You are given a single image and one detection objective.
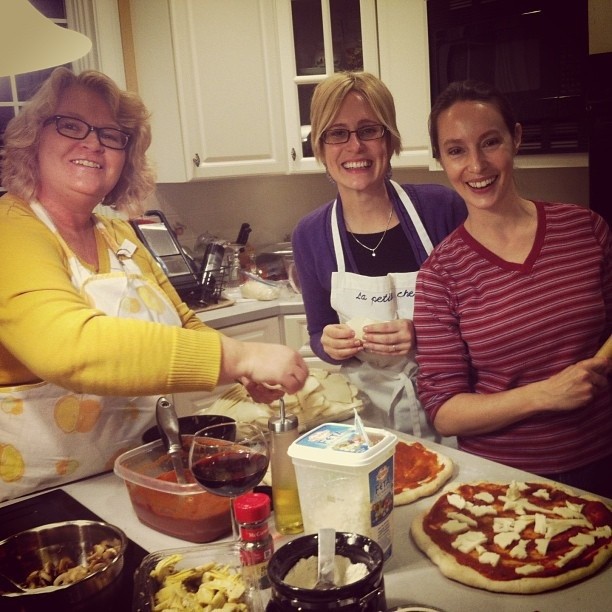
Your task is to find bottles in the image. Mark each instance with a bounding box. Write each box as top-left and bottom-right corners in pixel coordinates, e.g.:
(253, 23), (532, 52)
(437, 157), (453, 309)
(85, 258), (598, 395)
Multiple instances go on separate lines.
(230, 493), (275, 588)
(267, 396), (303, 536)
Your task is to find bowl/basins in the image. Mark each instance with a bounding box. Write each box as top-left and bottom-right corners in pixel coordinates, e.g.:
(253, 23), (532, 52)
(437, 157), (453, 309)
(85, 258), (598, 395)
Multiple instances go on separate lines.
(1, 519), (128, 611)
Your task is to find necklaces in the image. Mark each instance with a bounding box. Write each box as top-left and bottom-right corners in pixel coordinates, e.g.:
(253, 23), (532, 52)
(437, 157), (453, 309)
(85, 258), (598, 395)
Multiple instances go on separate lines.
(343, 204), (395, 256)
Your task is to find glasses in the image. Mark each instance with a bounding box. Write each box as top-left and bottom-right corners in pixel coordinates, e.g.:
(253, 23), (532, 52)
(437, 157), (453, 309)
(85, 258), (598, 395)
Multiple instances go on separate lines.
(319, 125), (390, 144)
(43, 115), (133, 150)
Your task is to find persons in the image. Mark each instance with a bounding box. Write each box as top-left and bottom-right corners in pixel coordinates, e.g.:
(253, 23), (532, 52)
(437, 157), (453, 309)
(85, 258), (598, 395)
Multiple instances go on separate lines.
(1, 63), (310, 502)
(289, 71), (467, 452)
(412, 83), (610, 503)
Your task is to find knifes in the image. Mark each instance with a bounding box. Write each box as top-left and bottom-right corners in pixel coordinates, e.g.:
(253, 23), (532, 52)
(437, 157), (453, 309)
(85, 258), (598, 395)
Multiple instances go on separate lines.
(240, 227), (251, 243)
(235, 222), (250, 244)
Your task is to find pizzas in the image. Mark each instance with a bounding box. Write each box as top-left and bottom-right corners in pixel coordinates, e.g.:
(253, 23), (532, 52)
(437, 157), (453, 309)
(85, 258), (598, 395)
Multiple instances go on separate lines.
(393, 438), (454, 507)
(409, 479), (612, 594)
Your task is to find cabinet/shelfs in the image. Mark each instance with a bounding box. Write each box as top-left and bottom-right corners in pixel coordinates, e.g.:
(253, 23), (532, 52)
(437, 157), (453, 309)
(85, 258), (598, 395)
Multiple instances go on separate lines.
(216, 316), (282, 349)
(282, 313), (313, 356)
(276, 0), (431, 176)
(119, 1), (290, 183)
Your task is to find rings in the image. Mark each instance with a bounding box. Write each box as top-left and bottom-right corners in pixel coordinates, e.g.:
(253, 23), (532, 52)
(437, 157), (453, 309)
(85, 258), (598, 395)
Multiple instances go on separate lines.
(393, 345), (397, 352)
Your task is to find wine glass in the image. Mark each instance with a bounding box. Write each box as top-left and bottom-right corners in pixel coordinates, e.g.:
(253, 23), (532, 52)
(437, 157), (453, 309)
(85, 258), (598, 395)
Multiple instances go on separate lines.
(186, 419), (270, 542)
